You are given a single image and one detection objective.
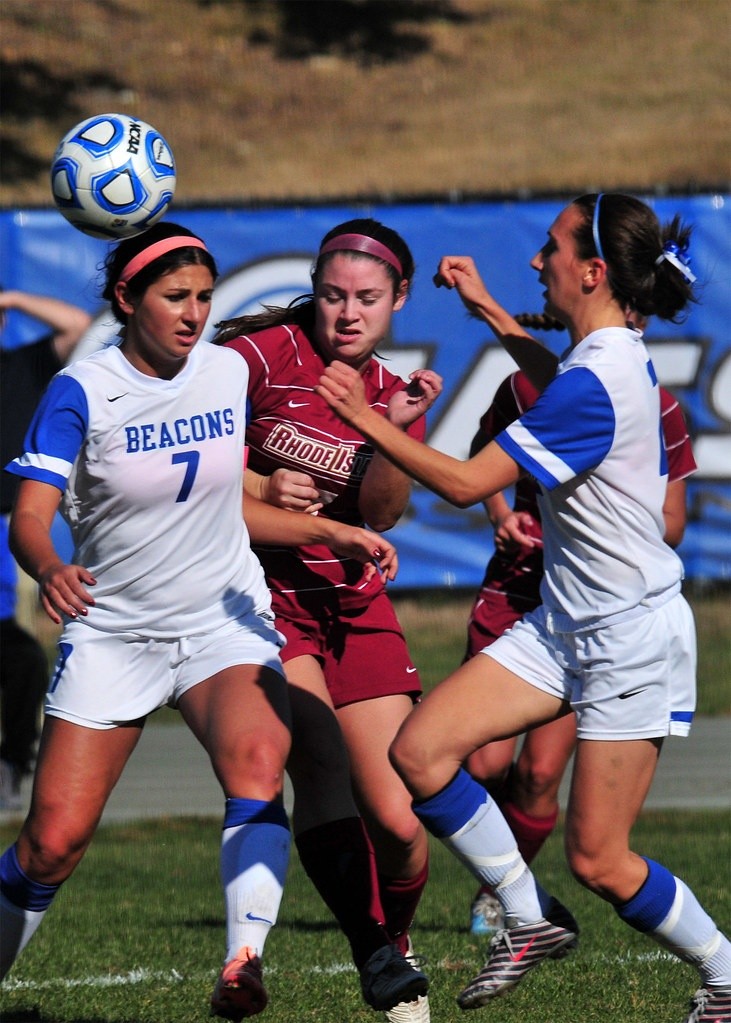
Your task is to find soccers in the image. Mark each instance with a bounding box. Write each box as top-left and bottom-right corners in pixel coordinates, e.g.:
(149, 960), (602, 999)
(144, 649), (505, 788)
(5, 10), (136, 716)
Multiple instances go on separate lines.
(49, 113), (178, 242)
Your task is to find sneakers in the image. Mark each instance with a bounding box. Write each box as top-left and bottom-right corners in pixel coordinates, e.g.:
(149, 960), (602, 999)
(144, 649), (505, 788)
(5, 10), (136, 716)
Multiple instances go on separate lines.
(455, 918), (577, 1010)
(469, 893), (506, 933)
(358, 934), (431, 1022)
(206, 946), (268, 1022)
(686, 984), (731, 1023)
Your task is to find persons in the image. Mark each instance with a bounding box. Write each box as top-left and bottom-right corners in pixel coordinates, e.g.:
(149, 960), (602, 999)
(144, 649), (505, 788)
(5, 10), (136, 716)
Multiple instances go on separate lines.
(0, 291), (93, 794)
(0, 220), (297, 1020)
(316, 191), (731, 1023)
(458, 303), (697, 937)
(209, 220), (443, 1023)
